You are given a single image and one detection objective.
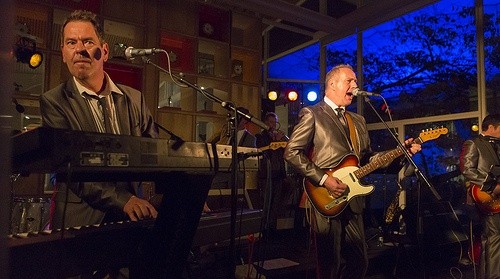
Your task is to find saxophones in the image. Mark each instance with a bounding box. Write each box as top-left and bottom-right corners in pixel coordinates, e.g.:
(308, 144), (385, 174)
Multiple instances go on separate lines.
(379, 176), (404, 225)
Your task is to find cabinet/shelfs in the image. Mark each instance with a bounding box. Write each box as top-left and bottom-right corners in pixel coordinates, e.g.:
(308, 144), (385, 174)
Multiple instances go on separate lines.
(156, 0), (263, 142)
(14, 0), (148, 199)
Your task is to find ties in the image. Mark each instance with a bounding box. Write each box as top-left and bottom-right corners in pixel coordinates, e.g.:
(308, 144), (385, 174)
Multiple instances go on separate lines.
(92, 95), (115, 135)
(335, 108), (354, 149)
(489, 141), (500, 161)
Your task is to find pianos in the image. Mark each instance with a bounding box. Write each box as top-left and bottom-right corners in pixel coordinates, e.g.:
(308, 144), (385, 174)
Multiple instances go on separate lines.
(0, 123), (273, 279)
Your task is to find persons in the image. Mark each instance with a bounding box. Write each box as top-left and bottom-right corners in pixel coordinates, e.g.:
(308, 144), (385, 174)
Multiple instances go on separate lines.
(255, 112), (288, 243)
(283, 64), (421, 279)
(39, 9), (209, 230)
(463, 113), (500, 279)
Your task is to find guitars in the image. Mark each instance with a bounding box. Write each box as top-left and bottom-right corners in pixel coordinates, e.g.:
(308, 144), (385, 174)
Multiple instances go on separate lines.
(248, 141), (292, 156)
(303, 126), (449, 218)
(471, 161), (500, 215)
(467, 236), (485, 266)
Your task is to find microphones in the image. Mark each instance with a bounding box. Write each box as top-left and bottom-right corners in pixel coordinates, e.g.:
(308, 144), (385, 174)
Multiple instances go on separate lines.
(124, 47), (165, 59)
(483, 136), (500, 141)
(352, 88), (380, 96)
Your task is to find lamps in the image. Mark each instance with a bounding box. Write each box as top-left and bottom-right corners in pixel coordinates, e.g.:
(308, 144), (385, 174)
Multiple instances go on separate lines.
(15, 45), (44, 69)
(266, 87), (281, 103)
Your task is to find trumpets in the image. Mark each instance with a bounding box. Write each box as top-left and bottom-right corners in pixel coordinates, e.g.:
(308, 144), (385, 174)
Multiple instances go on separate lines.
(271, 121), (295, 144)
